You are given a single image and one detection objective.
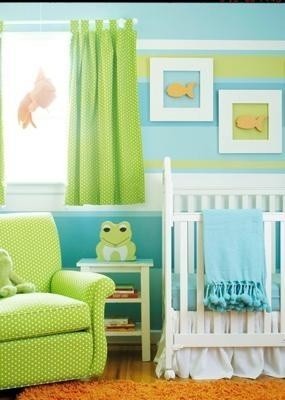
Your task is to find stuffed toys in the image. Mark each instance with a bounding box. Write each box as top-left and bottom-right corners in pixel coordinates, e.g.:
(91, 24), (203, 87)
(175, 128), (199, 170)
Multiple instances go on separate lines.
(96, 221), (138, 261)
(16, 70), (56, 129)
(0, 249), (36, 297)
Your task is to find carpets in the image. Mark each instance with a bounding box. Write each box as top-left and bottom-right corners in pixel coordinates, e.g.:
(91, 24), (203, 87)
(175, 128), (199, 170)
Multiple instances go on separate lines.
(14, 379), (285, 400)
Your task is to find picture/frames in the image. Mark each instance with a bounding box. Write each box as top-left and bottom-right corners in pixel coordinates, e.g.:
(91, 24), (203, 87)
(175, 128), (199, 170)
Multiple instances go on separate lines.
(150, 58), (283, 154)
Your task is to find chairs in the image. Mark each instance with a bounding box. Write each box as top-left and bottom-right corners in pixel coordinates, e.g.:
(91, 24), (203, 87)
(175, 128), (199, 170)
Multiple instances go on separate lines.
(0, 212), (115, 392)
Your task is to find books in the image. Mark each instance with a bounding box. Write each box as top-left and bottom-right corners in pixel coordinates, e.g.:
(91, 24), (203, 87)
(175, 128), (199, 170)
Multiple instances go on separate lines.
(106, 286), (139, 298)
(104, 314), (137, 332)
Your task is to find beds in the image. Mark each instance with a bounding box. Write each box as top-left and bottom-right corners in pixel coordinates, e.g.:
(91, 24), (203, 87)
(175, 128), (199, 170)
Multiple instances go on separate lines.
(161, 157), (285, 380)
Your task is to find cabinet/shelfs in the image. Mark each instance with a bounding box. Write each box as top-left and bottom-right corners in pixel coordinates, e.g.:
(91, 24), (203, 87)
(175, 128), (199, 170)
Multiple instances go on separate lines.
(75, 258), (153, 362)
